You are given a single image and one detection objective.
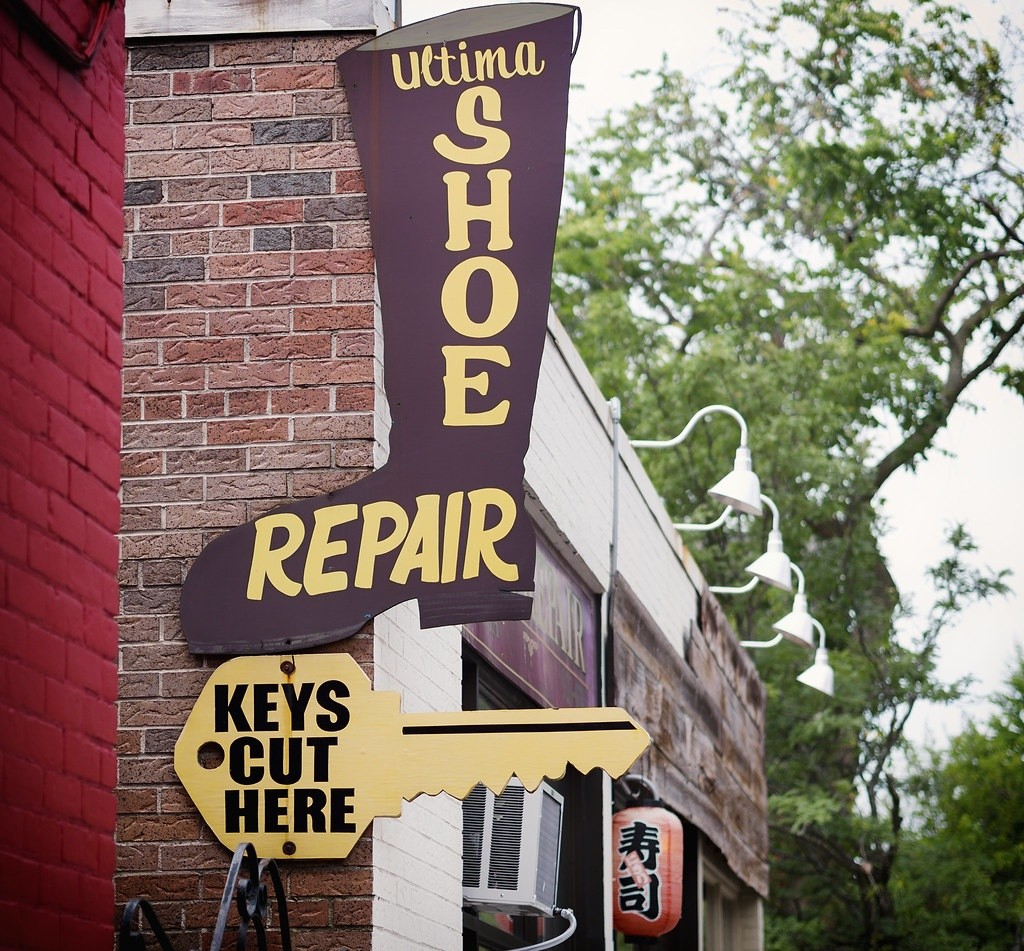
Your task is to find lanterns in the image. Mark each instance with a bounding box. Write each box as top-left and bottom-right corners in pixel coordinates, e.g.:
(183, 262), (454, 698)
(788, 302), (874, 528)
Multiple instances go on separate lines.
(612, 807), (684, 942)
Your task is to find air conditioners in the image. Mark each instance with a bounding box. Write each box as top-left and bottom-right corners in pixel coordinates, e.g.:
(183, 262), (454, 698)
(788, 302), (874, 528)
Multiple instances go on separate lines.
(462, 776), (565, 918)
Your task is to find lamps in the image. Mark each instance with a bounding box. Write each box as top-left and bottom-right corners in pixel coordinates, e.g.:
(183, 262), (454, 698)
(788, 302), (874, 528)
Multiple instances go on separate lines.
(628, 404), (835, 699)
